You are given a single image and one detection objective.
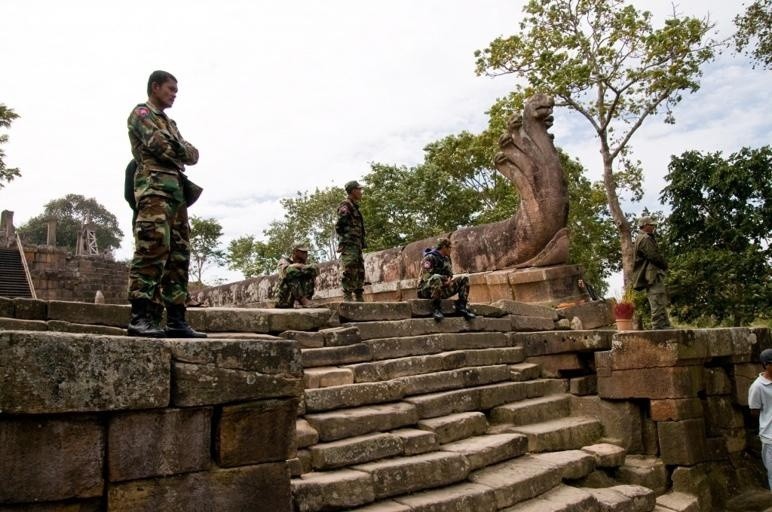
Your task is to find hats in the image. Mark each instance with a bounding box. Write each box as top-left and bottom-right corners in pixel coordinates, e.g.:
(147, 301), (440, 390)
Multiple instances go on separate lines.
(292, 241), (310, 252)
(437, 237), (453, 248)
(345, 180), (364, 191)
(760, 348), (772, 367)
(639, 217), (657, 227)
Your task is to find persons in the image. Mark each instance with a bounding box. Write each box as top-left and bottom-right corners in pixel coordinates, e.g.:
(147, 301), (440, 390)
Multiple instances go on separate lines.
(274, 241), (323, 308)
(630, 215), (675, 329)
(416, 237), (476, 319)
(335, 180), (368, 301)
(747, 347), (772, 490)
(126, 69), (208, 338)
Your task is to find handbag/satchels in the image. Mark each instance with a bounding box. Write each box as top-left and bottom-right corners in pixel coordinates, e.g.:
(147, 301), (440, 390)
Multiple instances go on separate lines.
(179, 172), (203, 207)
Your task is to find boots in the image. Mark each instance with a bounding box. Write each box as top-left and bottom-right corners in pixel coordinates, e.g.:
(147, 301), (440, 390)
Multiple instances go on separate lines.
(431, 298), (445, 319)
(455, 299), (475, 320)
(125, 297), (208, 339)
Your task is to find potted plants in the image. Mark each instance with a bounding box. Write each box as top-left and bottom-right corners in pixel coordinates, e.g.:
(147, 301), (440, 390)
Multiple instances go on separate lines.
(611, 301), (634, 330)
